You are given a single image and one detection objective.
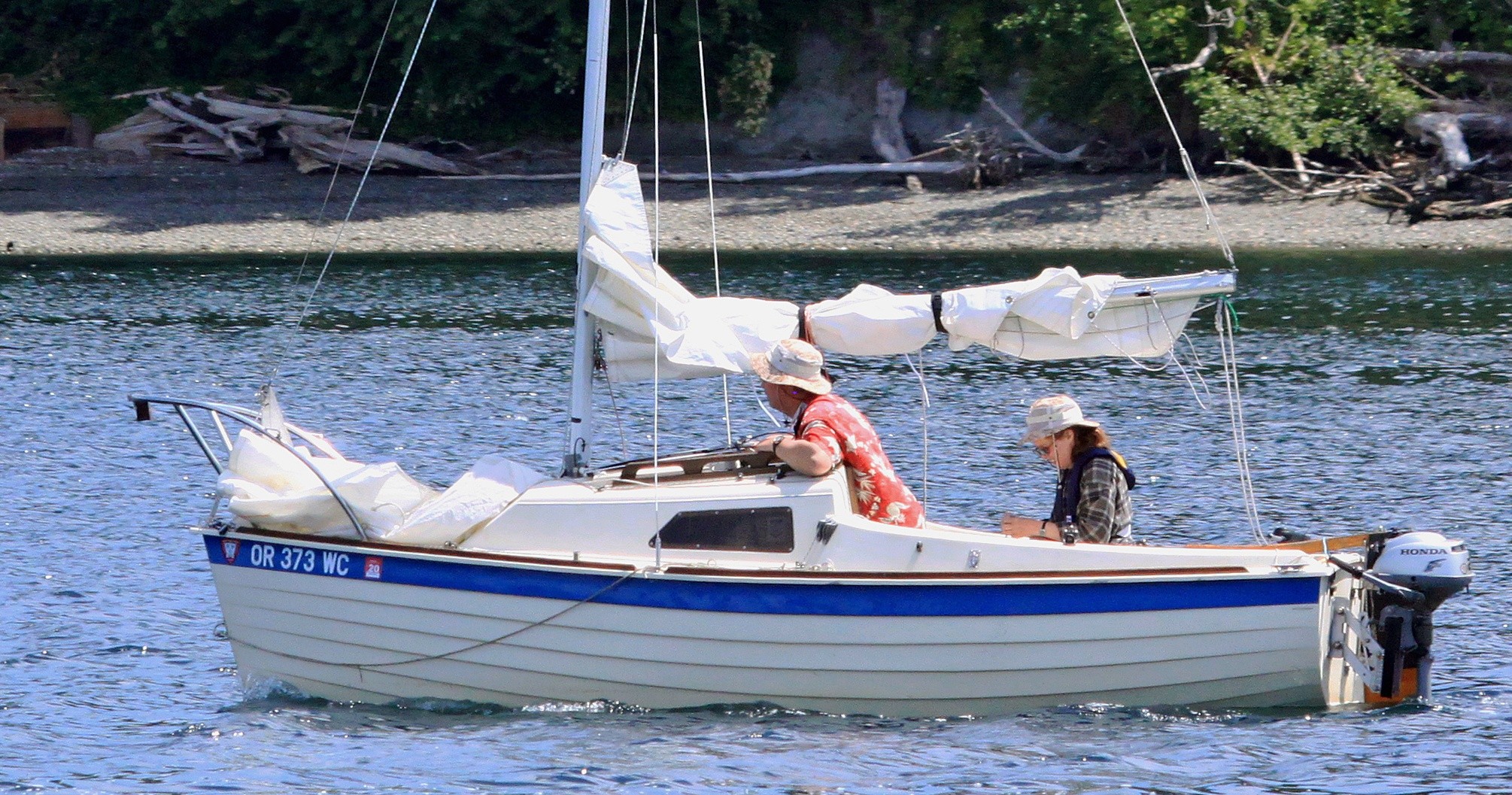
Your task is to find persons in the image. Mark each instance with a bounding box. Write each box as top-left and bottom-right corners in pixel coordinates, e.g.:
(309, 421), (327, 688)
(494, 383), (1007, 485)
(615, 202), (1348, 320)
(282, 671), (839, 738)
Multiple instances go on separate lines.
(752, 337), (925, 529)
(1000, 395), (1133, 544)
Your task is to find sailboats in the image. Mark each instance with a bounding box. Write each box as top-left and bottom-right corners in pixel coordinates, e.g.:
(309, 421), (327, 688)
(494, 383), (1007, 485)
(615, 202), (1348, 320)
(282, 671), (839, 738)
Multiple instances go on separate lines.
(127, 0), (1475, 715)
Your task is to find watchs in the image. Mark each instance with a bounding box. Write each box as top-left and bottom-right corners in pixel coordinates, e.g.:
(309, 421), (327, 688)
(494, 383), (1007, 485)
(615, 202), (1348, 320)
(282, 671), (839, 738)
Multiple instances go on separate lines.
(773, 434), (785, 455)
(1039, 521), (1048, 537)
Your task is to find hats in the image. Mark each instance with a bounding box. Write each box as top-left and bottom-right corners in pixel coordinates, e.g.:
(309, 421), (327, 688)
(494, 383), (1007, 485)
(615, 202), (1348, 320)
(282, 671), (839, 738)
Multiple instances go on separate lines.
(748, 338), (832, 396)
(1017, 394), (1101, 446)
